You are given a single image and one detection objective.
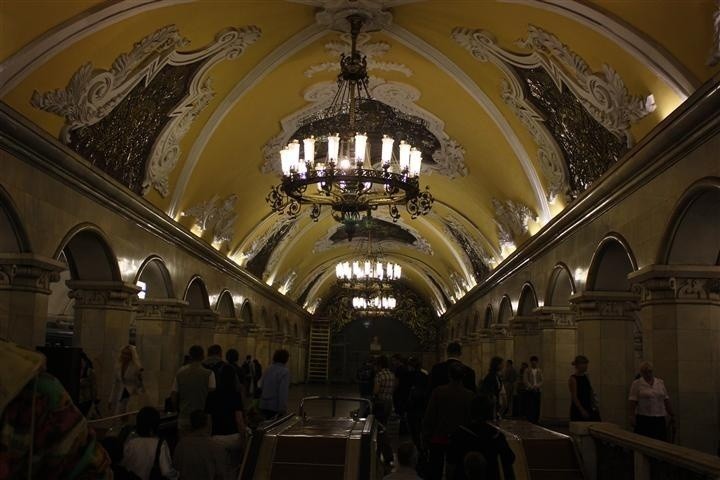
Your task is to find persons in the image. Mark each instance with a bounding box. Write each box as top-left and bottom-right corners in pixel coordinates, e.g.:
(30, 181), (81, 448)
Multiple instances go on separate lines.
(108, 343), (291, 480)
(625, 361), (675, 442)
(568, 356), (602, 422)
(358, 342), (543, 480)
